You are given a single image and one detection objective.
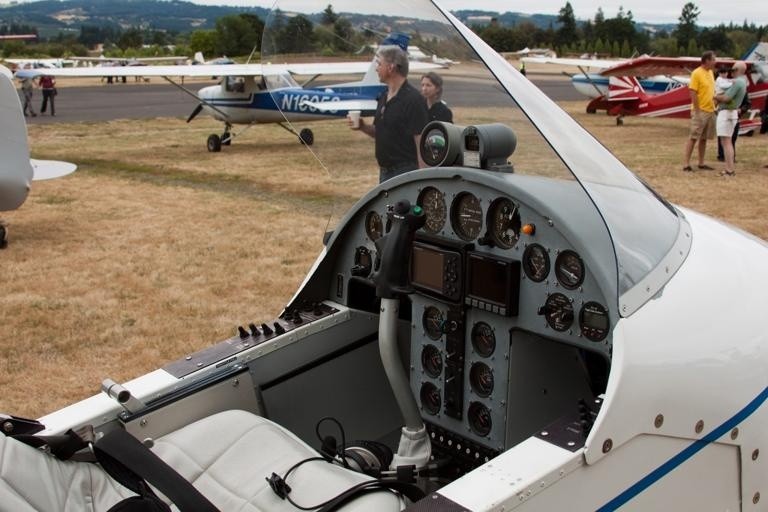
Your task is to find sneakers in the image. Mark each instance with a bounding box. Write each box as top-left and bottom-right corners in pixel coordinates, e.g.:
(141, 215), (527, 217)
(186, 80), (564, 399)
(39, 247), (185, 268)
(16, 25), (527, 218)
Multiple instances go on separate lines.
(684, 166), (692, 172)
(699, 164), (713, 169)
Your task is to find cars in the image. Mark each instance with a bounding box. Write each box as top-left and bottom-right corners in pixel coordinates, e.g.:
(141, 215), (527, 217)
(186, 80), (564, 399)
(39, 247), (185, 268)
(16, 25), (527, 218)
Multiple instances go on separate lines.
(7, 56), (73, 72)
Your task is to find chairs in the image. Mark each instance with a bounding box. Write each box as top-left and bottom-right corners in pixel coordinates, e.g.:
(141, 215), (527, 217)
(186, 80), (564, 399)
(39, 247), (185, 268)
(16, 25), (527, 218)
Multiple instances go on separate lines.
(0, 408), (407, 512)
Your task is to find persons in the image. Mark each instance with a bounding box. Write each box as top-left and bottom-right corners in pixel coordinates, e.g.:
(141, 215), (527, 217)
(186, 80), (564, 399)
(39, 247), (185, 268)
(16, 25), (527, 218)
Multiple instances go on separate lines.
(416, 71), (455, 125)
(99, 60), (141, 85)
(716, 92), (748, 164)
(37, 65), (58, 117)
(681, 51), (719, 172)
(713, 60), (748, 178)
(345, 44), (433, 185)
(18, 64), (39, 118)
(714, 66), (733, 116)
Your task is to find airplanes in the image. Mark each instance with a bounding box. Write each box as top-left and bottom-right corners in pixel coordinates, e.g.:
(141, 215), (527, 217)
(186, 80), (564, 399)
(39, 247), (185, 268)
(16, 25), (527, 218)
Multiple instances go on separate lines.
(581, 51), (768, 138)
(523, 40), (768, 99)
(75, 53), (190, 83)
(13, 54), (451, 152)
(0, 0), (768, 512)
(0, 31), (80, 248)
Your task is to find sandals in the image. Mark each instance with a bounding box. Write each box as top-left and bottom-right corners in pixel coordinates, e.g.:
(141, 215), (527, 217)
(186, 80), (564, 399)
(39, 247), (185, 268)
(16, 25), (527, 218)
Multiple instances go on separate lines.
(717, 170), (736, 177)
